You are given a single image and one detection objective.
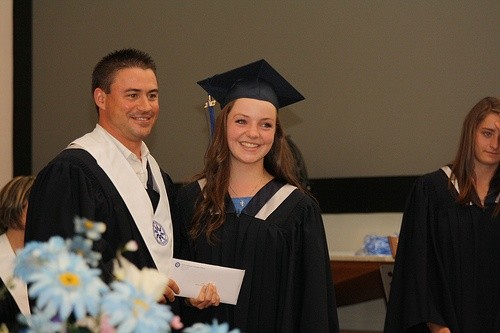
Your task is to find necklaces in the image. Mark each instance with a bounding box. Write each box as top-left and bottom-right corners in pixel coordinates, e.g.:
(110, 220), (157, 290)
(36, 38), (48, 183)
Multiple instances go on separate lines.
(226, 181), (264, 207)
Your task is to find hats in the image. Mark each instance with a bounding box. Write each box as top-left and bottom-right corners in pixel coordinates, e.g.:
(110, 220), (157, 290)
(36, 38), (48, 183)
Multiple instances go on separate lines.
(196, 59), (307, 111)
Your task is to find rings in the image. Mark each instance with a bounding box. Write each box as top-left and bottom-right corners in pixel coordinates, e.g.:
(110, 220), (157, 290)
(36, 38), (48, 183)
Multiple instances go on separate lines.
(196, 304), (204, 309)
(192, 303), (199, 307)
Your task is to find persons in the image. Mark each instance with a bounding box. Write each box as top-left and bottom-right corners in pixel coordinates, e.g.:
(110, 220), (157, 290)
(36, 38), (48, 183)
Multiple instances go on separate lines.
(384, 96), (500, 333)
(167, 58), (341, 333)
(0, 176), (38, 333)
(21, 47), (221, 333)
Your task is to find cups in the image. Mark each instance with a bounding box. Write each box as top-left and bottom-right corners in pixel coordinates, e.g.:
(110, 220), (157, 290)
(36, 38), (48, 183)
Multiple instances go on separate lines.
(387, 235), (398, 257)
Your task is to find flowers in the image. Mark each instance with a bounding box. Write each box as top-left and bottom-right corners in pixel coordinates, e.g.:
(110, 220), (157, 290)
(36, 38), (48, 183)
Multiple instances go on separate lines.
(6, 218), (240, 333)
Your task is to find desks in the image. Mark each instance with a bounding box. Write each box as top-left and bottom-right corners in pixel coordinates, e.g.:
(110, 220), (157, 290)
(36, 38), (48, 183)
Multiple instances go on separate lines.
(329, 253), (394, 306)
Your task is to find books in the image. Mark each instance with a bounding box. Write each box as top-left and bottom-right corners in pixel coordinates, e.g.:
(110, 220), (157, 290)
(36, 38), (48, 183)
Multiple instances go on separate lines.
(387, 235), (398, 259)
